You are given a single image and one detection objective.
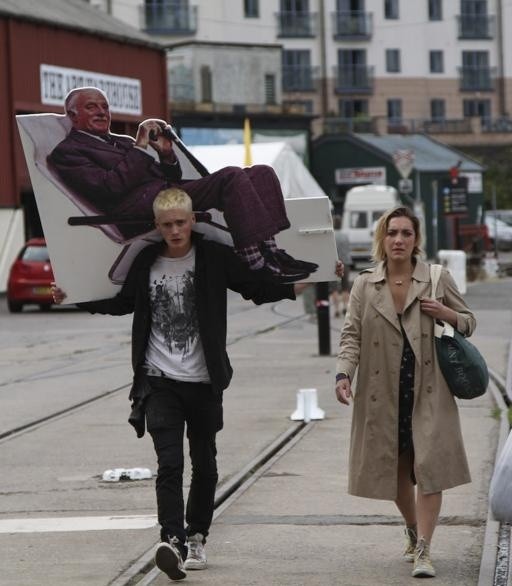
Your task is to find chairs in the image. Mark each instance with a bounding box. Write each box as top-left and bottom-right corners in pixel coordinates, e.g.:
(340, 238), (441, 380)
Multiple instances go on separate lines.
(14, 111), (280, 291)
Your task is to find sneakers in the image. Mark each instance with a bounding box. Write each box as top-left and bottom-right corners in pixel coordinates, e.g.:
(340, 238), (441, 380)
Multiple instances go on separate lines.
(403, 522), (435, 578)
(185, 533), (207, 569)
(153, 538), (187, 580)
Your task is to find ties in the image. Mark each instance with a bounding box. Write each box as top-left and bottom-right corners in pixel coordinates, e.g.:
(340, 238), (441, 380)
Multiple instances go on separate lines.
(101, 134), (130, 152)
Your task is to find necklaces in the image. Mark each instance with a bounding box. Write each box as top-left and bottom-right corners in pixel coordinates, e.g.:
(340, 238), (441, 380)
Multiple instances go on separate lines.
(394, 276), (413, 285)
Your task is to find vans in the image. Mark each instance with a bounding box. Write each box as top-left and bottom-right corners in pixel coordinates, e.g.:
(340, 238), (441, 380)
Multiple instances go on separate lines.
(341, 184), (402, 269)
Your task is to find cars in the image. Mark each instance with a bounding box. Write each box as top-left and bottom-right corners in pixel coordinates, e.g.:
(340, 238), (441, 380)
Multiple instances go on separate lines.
(5, 239), (56, 314)
(484, 213), (512, 252)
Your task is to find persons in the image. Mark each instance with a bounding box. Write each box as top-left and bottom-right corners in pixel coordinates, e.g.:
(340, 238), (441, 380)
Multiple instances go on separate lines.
(334, 205), (476, 579)
(46, 88), (319, 284)
(50, 187), (346, 581)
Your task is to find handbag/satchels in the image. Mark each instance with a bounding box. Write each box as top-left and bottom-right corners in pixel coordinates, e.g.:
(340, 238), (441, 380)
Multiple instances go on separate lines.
(434, 319), (488, 399)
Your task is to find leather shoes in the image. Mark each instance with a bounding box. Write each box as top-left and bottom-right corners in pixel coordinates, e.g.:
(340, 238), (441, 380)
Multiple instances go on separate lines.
(244, 249), (318, 283)
